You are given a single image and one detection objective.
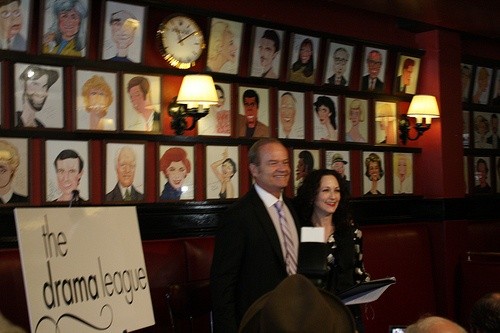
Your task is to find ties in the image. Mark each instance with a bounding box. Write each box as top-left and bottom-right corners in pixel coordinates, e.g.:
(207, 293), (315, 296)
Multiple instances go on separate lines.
(274, 201), (297, 276)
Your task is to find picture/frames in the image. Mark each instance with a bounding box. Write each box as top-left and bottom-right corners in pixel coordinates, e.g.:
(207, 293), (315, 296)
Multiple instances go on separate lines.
(460, 62), (500, 196)
(0, 0), (423, 206)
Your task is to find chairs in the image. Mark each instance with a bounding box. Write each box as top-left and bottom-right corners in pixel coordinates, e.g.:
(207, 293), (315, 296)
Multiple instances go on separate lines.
(165, 281), (213, 333)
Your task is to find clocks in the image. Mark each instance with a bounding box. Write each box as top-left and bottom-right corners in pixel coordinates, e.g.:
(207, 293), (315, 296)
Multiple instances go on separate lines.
(154, 15), (205, 69)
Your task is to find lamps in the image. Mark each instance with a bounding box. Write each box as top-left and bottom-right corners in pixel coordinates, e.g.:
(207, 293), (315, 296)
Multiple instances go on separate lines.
(399, 95), (440, 145)
(168, 73), (218, 135)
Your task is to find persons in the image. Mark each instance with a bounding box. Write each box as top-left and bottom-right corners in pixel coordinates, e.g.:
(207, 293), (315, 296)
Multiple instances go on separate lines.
(239, 274), (356, 333)
(468, 293), (500, 333)
(209, 137), (307, 333)
(406, 316), (467, 333)
(293, 168), (370, 333)
